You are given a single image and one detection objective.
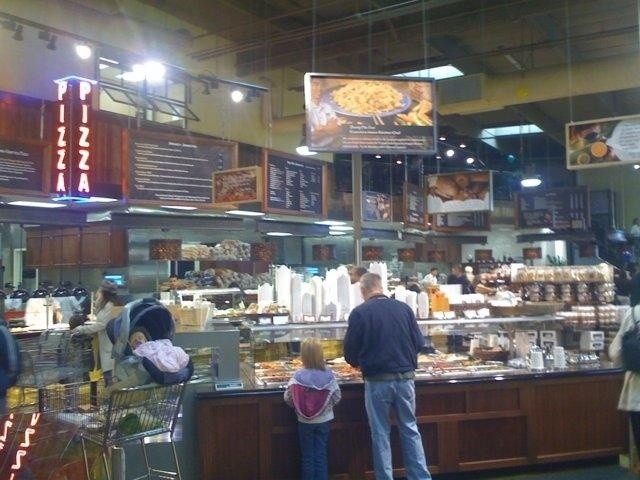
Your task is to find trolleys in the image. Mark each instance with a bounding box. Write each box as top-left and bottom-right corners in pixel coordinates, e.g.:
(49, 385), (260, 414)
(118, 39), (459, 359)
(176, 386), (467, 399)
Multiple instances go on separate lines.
(0, 327), (189, 480)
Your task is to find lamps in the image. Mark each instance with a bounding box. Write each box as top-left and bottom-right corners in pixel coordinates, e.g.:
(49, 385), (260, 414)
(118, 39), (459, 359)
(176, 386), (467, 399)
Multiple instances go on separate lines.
(522, 241), (542, 260)
(1, 10), (269, 103)
(251, 234), (279, 261)
(578, 240), (599, 258)
(427, 244), (446, 263)
(312, 236), (337, 261)
(474, 244), (493, 261)
(521, 124), (541, 188)
(362, 238), (384, 261)
(398, 242), (416, 261)
(148, 226), (181, 261)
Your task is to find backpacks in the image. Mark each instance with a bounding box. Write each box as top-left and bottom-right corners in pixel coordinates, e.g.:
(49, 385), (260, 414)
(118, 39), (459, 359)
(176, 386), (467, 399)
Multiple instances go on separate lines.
(623, 310), (640, 371)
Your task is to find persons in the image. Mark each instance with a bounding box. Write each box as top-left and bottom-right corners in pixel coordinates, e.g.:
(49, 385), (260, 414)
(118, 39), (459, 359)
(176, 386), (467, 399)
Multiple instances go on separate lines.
(422, 267), (438, 284)
(608, 270), (640, 477)
(348, 266), (370, 308)
(0, 278), (125, 409)
(310, 74), (345, 149)
(631, 218), (640, 237)
(444, 262), (476, 353)
(0, 292), (23, 416)
(219, 282), (261, 343)
(343, 271), (432, 480)
(284, 336), (343, 479)
(163, 272), (190, 301)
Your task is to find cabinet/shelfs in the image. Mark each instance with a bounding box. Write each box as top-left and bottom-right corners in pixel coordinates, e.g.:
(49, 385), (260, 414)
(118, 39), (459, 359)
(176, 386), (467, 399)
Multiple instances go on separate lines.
(23, 225), (127, 271)
(193, 316), (629, 480)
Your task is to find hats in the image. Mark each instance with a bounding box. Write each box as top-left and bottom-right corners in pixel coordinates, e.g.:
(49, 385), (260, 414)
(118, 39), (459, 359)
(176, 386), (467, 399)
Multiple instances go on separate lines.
(100, 280), (118, 294)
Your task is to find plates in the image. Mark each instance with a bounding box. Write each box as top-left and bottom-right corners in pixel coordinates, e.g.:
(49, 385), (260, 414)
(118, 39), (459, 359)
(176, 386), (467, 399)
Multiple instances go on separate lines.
(321, 83), (413, 119)
(577, 153), (591, 165)
(591, 141), (608, 158)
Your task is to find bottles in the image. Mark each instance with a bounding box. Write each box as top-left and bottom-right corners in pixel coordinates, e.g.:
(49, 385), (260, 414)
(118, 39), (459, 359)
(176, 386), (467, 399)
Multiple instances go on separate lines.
(0, 281), (90, 314)
(477, 259), (496, 274)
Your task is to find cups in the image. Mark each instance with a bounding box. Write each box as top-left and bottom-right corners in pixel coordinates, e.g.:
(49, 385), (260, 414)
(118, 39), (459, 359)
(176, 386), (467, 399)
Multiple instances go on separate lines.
(553, 347), (565, 367)
(258, 260), (431, 323)
(530, 352), (544, 370)
(490, 335), (498, 347)
(470, 339), (480, 354)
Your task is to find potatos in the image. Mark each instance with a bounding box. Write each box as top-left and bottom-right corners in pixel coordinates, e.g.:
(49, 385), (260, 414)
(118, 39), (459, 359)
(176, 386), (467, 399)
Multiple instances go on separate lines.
(436, 173), (489, 201)
